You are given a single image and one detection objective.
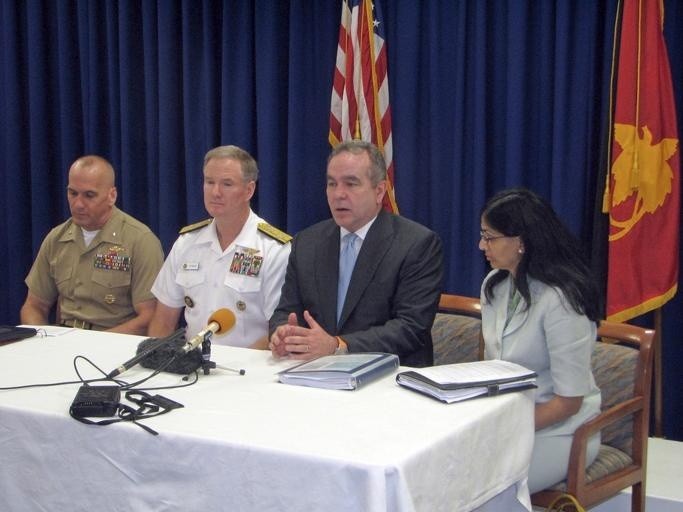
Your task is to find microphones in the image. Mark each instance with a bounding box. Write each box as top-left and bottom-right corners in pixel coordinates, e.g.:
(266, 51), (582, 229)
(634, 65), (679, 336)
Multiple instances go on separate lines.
(174, 308), (235, 360)
(107, 327), (186, 377)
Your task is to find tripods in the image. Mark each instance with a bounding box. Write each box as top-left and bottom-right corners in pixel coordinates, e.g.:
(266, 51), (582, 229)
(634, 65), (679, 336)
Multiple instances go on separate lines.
(183, 343), (245, 380)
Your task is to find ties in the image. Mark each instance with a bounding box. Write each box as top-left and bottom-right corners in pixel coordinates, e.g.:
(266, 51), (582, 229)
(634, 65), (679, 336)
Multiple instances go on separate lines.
(335, 233), (359, 325)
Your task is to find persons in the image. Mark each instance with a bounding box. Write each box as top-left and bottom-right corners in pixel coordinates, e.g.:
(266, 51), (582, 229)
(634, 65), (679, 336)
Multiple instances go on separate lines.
(19, 154), (165, 335)
(268, 141), (444, 368)
(147, 145), (292, 350)
(478, 188), (601, 493)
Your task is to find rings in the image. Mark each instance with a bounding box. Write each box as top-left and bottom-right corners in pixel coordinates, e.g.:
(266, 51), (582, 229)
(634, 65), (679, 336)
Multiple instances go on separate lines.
(307, 345), (309, 351)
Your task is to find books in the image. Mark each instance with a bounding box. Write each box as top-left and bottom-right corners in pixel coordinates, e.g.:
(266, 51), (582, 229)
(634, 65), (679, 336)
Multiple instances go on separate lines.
(273, 352), (539, 404)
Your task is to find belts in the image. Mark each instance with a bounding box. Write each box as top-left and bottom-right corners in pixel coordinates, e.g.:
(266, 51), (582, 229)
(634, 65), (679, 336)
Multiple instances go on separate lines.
(64, 318), (109, 332)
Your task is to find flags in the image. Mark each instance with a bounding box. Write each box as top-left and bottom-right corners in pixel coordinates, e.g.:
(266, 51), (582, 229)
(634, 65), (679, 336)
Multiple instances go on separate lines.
(601, 0), (681, 323)
(328, 1), (401, 216)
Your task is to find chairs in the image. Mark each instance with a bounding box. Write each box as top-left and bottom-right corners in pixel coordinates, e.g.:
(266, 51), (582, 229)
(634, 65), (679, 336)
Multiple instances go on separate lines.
(430, 294), (486, 376)
(530, 325), (658, 512)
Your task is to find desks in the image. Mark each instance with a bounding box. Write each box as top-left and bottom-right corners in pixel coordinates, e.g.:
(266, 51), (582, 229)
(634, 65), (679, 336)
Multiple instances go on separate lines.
(0, 324), (535, 512)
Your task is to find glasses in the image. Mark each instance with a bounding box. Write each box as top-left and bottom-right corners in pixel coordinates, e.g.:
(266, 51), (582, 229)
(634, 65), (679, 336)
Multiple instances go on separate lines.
(480, 230), (506, 244)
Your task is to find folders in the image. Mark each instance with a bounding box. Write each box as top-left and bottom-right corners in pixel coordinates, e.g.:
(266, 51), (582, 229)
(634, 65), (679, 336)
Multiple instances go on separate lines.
(271, 347), (539, 407)
